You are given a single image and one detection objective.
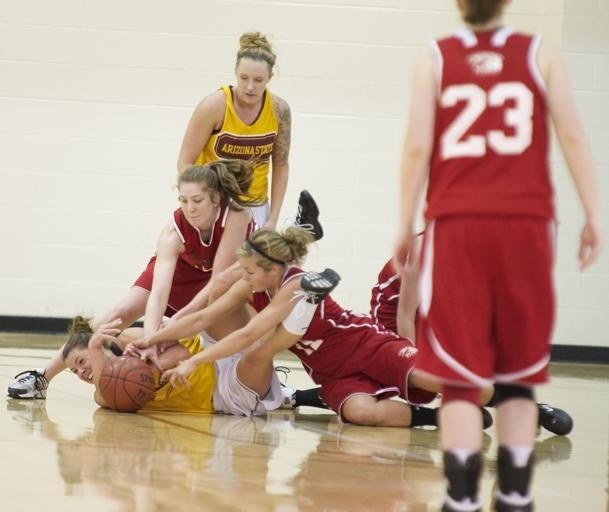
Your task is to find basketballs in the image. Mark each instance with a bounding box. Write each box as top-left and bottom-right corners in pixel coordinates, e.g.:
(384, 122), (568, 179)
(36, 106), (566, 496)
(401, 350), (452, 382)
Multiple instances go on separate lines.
(98, 355), (155, 411)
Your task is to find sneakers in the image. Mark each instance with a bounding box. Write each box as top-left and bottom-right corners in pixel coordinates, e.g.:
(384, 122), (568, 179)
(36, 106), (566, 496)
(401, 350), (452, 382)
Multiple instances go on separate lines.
(8, 367), (49, 399)
(278, 387), (296, 409)
(433, 405), (493, 429)
(538, 404), (573, 435)
(295, 191), (323, 241)
(300, 268), (340, 304)
(442, 483), (535, 512)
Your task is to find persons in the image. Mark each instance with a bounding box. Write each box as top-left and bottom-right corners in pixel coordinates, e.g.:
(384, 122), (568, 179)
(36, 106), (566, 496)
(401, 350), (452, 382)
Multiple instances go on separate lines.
(393, 0), (601, 512)
(276, 227), (425, 409)
(6, 160), (255, 400)
(122, 224), (575, 436)
(176, 31), (292, 231)
(61, 190), (341, 416)
